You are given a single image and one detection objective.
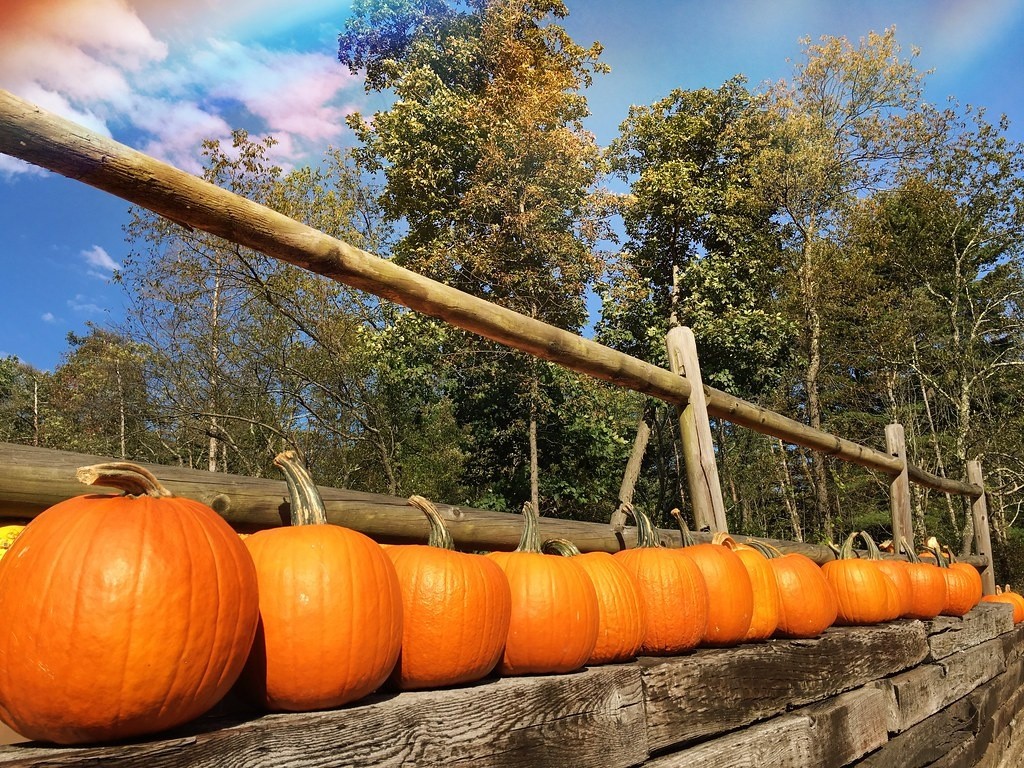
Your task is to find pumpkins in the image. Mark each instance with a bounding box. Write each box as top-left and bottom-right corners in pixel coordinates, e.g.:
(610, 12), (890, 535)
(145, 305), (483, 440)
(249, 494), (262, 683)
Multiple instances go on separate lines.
(885, 540), (1024, 625)
(0, 449), (902, 746)
